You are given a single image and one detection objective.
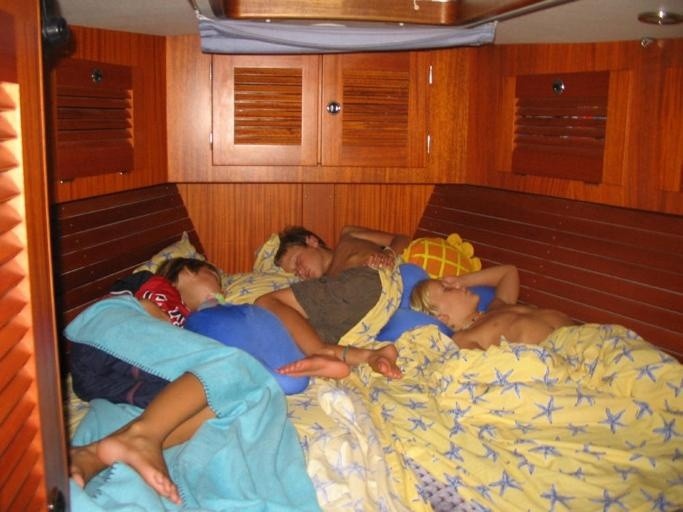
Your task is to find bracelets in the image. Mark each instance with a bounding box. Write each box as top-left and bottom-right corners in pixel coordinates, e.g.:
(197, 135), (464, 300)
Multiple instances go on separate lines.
(383, 246), (398, 256)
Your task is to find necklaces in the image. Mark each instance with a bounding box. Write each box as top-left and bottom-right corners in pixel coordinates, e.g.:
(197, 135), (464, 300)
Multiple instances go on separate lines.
(459, 309), (485, 333)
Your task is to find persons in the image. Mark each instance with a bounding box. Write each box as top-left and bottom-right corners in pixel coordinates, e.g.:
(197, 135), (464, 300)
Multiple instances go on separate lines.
(64, 258), (224, 501)
(410, 263), (579, 354)
(253, 227), (409, 381)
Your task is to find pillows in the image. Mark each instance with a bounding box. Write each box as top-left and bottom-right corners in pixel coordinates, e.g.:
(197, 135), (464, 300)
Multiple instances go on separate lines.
(185, 300), (310, 395)
(400, 234), (482, 279)
(376, 263), (497, 343)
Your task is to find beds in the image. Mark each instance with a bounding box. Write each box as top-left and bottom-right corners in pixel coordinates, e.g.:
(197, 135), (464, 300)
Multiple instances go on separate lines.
(49, 182), (683, 512)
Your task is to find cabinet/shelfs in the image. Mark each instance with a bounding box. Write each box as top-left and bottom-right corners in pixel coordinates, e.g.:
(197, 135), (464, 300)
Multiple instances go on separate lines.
(42, 23), (168, 202)
(485, 40), (654, 210)
(209, 34), (430, 170)
